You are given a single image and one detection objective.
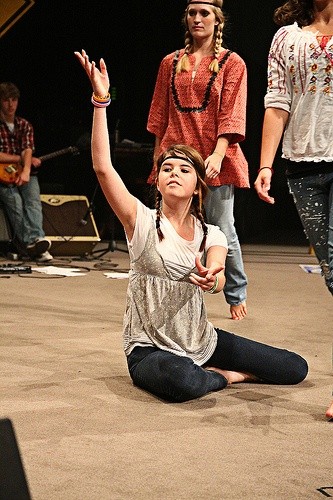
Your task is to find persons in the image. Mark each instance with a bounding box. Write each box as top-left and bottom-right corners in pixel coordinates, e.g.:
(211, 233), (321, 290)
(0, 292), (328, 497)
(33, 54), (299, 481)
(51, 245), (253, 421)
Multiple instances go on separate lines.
(0, 83), (54, 262)
(147, 0), (249, 321)
(254, 0), (333, 420)
(75, 49), (308, 403)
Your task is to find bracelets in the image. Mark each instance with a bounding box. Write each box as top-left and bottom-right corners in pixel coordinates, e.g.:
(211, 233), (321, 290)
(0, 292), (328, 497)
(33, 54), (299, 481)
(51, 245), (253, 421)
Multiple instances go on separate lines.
(213, 151), (225, 159)
(258, 166), (272, 173)
(207, 276), (218, 295)
(92, 92), (111, 108)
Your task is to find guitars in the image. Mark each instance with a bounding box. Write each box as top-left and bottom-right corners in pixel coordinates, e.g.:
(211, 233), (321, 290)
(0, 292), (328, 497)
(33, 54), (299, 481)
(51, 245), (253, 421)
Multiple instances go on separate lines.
(0, 146), (78, 184)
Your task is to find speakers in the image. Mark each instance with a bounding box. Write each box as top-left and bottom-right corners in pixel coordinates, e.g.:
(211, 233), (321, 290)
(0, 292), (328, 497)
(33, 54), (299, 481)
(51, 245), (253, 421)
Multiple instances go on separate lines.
(0, 194), (101, 256)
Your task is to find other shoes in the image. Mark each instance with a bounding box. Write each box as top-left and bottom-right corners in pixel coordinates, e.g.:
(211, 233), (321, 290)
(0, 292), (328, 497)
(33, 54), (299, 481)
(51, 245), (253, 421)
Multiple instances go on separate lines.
(28, 239), (53, 262)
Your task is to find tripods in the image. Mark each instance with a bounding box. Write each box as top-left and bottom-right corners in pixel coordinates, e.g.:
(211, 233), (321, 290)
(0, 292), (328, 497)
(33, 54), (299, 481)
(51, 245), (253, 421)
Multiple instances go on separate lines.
(90, 119), (129, 262)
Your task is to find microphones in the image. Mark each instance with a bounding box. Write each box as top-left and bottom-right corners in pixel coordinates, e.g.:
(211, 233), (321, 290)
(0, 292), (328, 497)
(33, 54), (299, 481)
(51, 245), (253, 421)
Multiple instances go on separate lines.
(80, 205), (93, 225)
